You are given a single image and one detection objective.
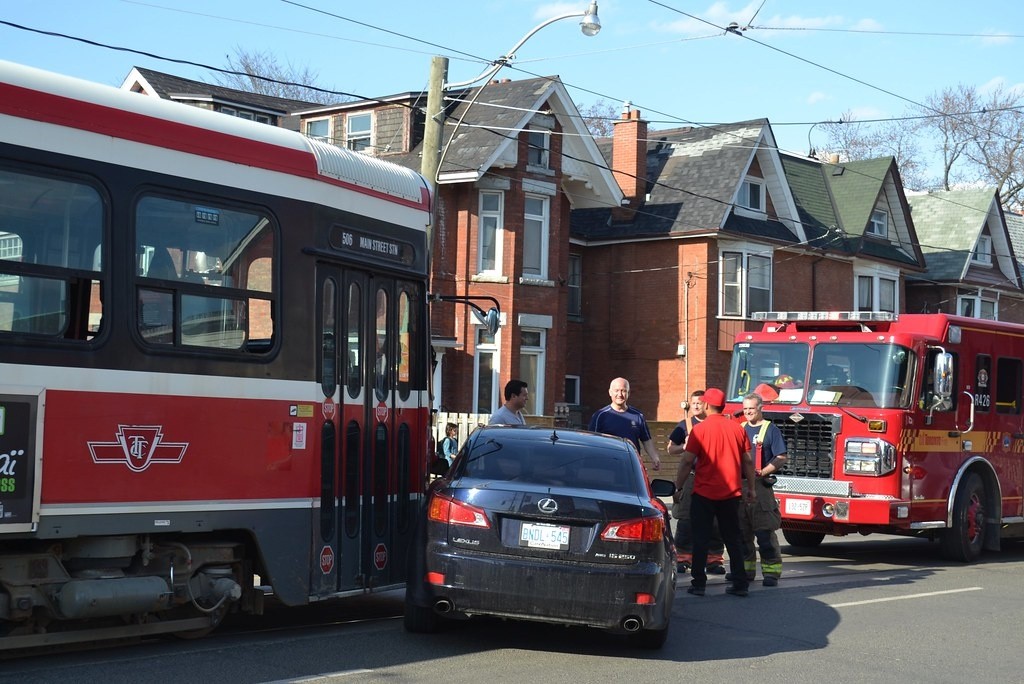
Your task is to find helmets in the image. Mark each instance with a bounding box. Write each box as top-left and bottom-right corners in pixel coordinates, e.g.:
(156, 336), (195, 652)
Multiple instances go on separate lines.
(775, 375), (802, 389)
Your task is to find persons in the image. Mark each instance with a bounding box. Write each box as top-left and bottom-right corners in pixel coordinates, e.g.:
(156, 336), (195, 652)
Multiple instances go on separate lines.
(489, 379), (529, 425)
(443, 423), (458, 466)
(588, 377), (660, 470)
(725, 393), (788, 587)
(667, 390), (726, 575)
(672, 387), (756, 597)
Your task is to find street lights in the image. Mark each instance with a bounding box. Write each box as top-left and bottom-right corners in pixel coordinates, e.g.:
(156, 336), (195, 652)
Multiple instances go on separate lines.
(415, 0), (601, 188)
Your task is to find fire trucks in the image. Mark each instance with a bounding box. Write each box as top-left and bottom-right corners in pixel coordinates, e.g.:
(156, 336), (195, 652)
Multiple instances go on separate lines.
(724, 309), (1024, 563)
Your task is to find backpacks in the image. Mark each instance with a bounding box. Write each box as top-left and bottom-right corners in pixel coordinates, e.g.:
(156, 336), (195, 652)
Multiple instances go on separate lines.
(437, 436), (459, 457)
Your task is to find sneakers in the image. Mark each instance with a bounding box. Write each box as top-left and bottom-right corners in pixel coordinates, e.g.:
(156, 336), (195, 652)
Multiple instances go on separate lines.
(724, 586), (748, 595)
(687, 584), (706, 595)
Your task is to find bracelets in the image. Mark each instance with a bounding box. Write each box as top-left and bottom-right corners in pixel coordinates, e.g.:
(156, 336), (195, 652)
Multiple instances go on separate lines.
(675, 488), (683, 493)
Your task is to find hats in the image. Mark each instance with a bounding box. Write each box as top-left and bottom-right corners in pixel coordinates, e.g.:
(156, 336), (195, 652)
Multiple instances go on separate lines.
(699, 388), (726, 407)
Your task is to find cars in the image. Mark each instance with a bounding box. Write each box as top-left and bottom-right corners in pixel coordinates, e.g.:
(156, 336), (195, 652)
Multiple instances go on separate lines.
(406, 421), (675, 653)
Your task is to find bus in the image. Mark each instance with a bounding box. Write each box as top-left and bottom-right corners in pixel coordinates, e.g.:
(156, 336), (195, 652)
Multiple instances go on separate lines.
(0, 60), (500, 640)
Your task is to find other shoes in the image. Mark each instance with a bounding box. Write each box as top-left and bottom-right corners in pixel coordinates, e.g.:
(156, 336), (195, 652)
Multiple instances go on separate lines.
(724, 572), (754, 581)
(676, 563), (687, 573)
(705, 562), (726, 574)
(762, 577), (778, 586)
